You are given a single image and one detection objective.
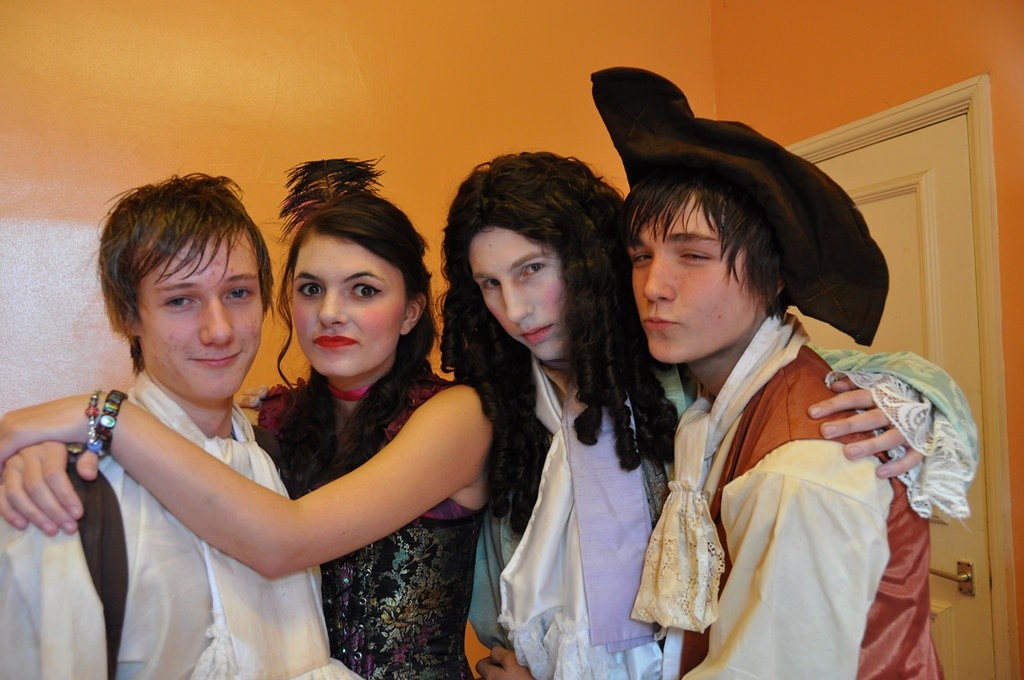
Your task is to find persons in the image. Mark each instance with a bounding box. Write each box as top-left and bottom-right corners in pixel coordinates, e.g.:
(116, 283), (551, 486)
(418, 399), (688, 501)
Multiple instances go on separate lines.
(0, 158), (493, 680)
(0, 173), (365, 680)
(476, 67), (945, 680)
(436, 153), (979, 680)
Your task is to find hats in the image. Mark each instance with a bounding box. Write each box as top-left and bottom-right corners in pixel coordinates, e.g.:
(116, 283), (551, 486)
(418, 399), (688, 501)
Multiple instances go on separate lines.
(588, 67), (889, 348)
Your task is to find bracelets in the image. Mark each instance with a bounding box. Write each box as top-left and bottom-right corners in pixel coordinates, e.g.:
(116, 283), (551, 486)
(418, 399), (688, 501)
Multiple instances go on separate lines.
(85, 388), (127, 458)
